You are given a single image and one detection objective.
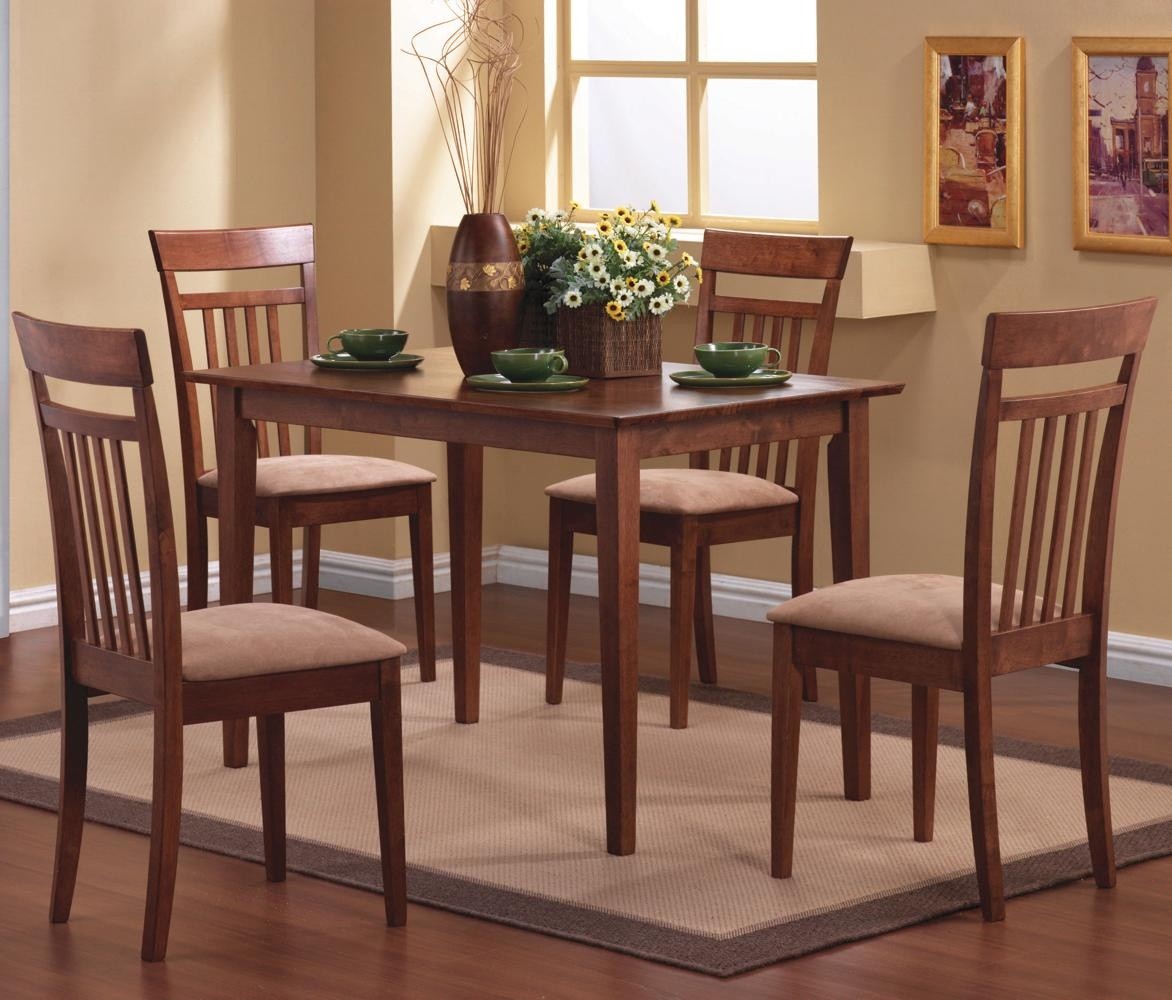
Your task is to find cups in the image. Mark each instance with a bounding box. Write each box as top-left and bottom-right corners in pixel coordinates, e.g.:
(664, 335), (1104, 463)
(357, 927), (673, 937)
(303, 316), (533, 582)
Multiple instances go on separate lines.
(327, 329), (411, 362)
(489, 347), (568, 382)
(693, 341), (782, 377)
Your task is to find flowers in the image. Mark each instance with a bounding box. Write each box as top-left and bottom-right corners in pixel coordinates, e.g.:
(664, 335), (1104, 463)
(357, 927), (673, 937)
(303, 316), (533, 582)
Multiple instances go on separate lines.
(513, 201), (703, 321)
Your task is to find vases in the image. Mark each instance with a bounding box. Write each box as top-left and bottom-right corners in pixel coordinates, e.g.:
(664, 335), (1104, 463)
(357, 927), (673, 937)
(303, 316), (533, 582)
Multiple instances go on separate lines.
(446, 213), (526, 376)
(518, 297), (662, 377)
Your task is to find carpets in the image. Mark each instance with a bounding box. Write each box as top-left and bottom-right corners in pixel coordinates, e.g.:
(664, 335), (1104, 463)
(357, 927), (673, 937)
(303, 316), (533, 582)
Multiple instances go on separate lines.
(0, 644), (1172, 976)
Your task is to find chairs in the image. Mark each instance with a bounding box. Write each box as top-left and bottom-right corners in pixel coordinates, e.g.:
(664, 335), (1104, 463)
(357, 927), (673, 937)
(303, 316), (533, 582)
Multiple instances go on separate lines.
(767, 296), (1158, 921)
(150, 223), (437, 681)
(544, 228), (854, 729)
(12, 311), (408, 963)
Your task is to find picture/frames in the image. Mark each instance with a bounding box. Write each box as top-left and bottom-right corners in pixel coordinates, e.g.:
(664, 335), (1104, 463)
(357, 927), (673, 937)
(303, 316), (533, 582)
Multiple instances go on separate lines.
(1072, 36), (1172, 257)
(918, 36), (1027, 248)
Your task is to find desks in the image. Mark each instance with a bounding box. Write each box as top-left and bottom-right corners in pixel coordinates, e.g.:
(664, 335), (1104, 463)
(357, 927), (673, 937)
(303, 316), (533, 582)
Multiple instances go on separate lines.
(182, 346), (908, 855)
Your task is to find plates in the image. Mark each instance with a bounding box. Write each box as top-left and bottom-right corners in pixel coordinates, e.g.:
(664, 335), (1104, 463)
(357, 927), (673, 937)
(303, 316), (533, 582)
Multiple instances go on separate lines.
(670, 370), (793, 389)
(466, 374), (591, 393)
(310, 353), (424, 372)
